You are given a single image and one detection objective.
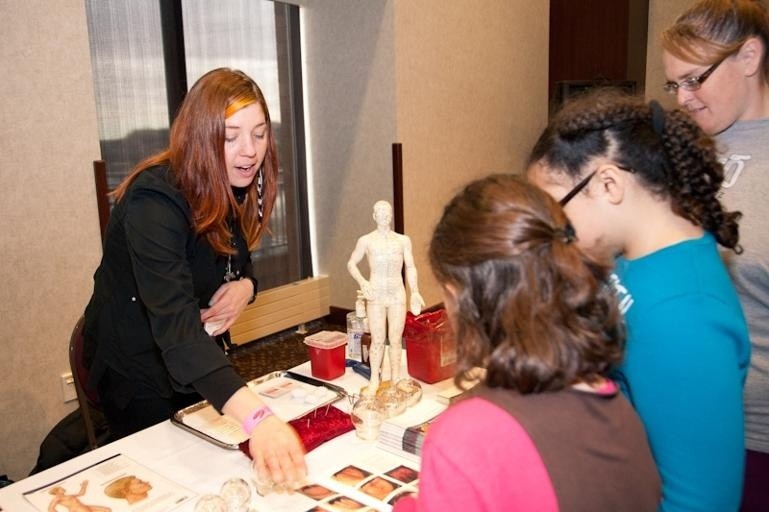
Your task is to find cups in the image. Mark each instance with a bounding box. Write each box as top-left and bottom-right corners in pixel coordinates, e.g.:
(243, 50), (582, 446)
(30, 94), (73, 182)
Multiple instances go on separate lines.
(305, 329), (347, 378)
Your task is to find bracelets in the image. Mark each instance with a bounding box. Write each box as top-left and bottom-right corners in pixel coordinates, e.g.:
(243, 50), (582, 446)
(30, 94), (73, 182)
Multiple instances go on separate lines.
(241, 406), (274, 437)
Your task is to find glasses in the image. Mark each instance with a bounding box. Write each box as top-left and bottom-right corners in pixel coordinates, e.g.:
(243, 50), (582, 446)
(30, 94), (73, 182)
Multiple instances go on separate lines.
(662, 59), (724, 95)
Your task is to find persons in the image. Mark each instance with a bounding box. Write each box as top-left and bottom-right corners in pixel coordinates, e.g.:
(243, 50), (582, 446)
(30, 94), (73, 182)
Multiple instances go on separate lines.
(526, 89), (752, 511)
(70, 69), (310, 494)
(347, 198), (427, 401)
(392, 173), (661, 511)
(662, 0), (769, 511)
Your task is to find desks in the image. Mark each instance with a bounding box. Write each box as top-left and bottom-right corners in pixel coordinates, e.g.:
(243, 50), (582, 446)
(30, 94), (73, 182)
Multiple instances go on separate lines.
(0, 338), (467, 512)
